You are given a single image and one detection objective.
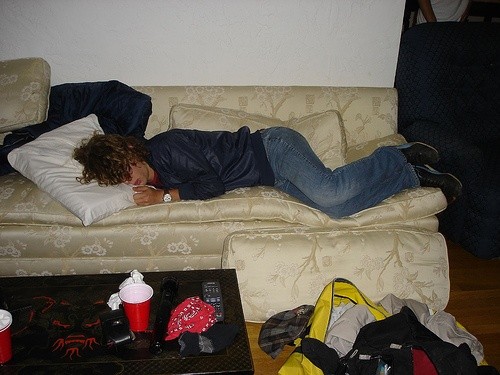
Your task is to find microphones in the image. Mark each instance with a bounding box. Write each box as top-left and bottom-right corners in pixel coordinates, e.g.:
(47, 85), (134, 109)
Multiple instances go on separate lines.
(148, 277), (179, 354)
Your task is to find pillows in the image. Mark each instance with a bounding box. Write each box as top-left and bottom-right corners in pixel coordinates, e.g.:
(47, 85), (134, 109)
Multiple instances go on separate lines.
(7, 114), (157, 227)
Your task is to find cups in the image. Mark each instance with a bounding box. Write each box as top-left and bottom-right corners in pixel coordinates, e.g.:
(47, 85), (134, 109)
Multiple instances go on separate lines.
(0, 309), (13, 364)
(119, 282), (154, 331)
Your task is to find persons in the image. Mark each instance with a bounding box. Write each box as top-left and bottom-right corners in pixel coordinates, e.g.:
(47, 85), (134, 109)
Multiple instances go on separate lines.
(73, 126), (462, 219)
(416, 0), (473, 24)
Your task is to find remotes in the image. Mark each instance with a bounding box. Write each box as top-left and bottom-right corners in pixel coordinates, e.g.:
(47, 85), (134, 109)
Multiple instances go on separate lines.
(202, 281), (224, 323)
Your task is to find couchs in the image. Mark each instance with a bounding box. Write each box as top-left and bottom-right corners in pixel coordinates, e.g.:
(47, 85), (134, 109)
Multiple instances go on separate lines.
(396, 21), (500, 261)
(0, 86), (439, 278)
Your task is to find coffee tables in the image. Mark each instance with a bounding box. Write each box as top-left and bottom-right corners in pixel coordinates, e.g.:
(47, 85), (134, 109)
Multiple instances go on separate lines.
(0, 269), (255, 375)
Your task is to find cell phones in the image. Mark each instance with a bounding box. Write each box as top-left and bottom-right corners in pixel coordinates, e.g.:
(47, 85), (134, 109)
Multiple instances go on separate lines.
(100, 308), (135, 347)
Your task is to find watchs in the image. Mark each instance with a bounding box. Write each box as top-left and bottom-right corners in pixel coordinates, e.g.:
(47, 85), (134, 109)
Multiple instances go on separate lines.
(162, 189), (172, 202)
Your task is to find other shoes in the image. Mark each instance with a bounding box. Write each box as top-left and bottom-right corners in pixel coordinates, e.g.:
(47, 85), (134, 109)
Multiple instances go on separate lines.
(387, 142), (439, 164)
(412, 164), (461, 196)
